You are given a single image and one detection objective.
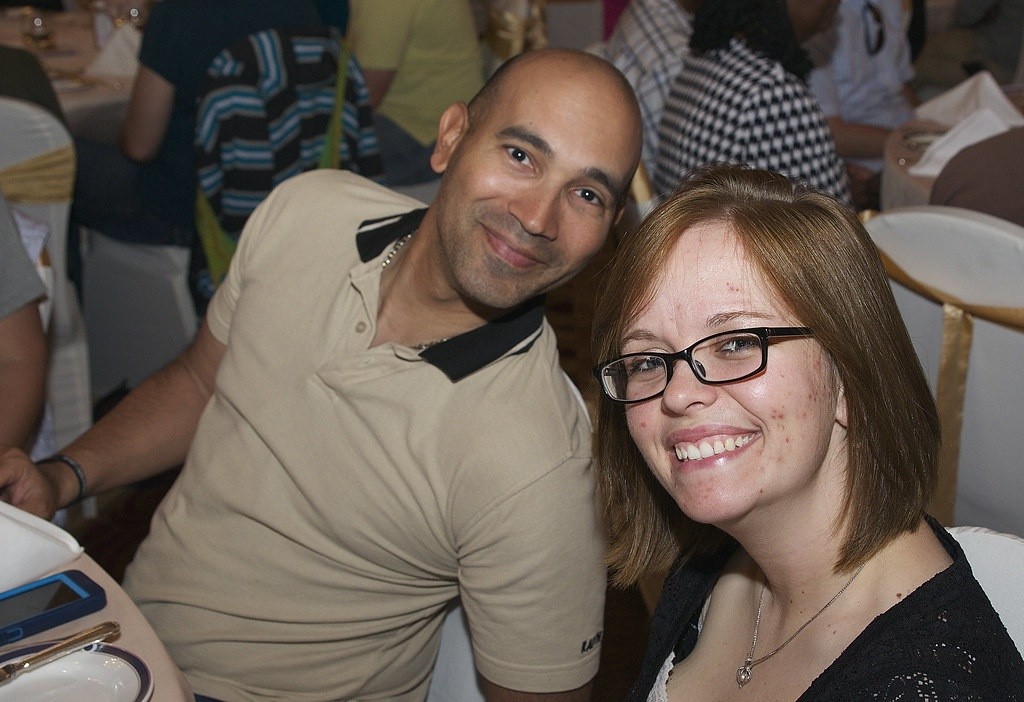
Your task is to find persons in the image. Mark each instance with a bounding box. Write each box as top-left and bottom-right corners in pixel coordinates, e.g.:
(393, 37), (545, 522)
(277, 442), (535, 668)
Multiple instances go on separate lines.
(0, 45), (643, 702)
(585, 170), (1024, 702)
(600, 1), (1024, 223)
(0, 0), (489, 457)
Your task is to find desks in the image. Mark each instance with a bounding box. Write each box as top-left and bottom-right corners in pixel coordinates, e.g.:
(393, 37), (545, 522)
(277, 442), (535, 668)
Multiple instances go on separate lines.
(0, 499), (196, 702)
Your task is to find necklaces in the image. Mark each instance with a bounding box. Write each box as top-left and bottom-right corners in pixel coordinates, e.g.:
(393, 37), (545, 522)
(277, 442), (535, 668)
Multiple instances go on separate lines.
(737, 561), (868, 685)
(383, 233), (451, 348)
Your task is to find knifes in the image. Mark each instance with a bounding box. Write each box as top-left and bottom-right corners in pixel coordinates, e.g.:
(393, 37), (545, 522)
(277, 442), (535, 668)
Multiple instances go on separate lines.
(0, 621), (119, 685)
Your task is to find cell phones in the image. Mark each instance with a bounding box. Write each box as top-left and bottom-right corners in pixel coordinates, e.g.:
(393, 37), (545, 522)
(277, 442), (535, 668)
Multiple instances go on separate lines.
(0, 570), (107, 647)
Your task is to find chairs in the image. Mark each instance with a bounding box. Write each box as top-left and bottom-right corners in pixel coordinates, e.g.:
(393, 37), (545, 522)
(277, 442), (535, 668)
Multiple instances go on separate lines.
(75, 146), (198, 420)
(0, 97), (78, 332)
(861, 205), (1024, 539)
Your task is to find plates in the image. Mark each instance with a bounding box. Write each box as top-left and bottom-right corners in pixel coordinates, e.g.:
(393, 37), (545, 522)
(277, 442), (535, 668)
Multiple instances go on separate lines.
(0, 642), (153, 702)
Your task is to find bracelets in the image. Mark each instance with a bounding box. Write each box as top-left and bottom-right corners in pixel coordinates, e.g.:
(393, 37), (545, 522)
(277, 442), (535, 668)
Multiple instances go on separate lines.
(39, 456), (85, 508)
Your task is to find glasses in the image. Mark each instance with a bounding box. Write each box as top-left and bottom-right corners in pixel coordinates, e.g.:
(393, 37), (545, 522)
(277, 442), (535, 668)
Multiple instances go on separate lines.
(591, 326), (814, 405)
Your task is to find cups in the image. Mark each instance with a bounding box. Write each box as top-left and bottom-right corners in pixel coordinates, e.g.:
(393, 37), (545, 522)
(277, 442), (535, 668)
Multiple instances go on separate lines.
(112, 0), (148, 34)
(20, 8), (53, 53)
